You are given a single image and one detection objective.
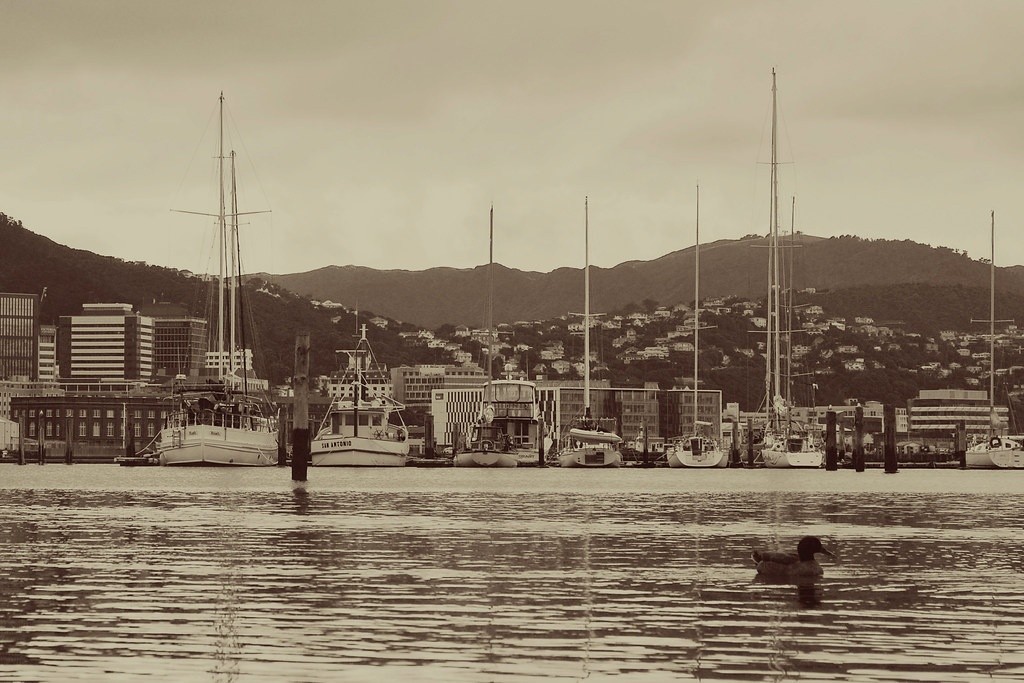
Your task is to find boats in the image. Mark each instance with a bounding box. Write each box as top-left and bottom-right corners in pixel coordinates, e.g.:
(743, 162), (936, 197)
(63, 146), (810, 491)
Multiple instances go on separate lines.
(568, 427), (623, 443)
(309, 296), (410, 467)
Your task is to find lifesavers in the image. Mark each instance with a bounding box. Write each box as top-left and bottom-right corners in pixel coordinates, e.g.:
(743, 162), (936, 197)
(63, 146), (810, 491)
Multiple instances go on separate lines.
(989, 437), (1002, 448)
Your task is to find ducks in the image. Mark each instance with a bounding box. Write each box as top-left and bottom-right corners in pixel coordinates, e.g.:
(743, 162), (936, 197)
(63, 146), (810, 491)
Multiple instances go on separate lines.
(751, 536), (837, 576)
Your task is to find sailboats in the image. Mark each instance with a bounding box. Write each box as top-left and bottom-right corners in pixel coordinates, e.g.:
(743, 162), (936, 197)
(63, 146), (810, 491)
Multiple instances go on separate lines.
(963, 210), (1024, 471)
(147, 90), (282, 468)
(449, 204), (521, 469)
(663, 179), (731, 468)
(742, 62), (828, 468)
(555, 194), (624, 470)
